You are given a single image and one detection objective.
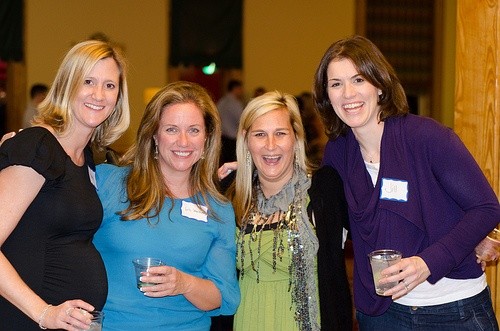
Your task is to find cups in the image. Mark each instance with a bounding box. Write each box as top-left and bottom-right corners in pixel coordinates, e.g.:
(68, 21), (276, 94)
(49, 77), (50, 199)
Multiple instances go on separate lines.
(87, 311), (104, 331)
(368, 249), (402, 296)
(132, 259), (166, 289)
